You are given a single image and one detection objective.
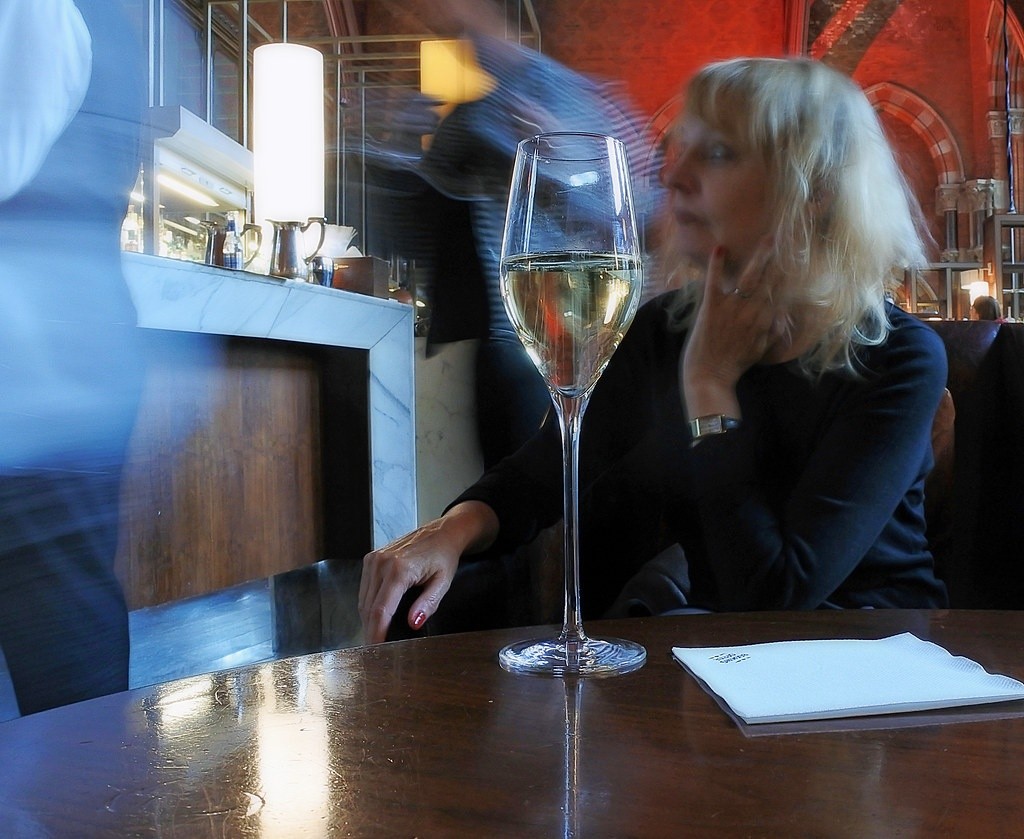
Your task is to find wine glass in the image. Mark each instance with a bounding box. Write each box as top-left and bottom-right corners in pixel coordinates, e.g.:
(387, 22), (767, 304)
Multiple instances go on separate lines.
(498, 131), (648, 678)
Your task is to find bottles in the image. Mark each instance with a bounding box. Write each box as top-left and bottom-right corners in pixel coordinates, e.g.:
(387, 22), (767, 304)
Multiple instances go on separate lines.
(125, 203), (141, 249)
(223, 231), (236, 269)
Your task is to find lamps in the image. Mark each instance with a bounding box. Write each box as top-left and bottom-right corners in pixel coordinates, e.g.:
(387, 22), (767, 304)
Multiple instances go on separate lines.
(253, 43), (327, 281)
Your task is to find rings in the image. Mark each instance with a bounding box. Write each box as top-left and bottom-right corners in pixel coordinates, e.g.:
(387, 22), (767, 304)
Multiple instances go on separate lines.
(734, 288), (752, 298)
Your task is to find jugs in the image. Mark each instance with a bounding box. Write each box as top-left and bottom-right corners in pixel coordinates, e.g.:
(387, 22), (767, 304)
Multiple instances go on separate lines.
(266, 217), (327, 282)
(200, 219), (262, 269)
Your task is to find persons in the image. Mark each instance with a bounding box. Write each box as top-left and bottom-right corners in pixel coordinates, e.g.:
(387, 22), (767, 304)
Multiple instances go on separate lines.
(969, 295), (1001, 319)
(0, 0), (144, 716)
(358, 58), (949, 649)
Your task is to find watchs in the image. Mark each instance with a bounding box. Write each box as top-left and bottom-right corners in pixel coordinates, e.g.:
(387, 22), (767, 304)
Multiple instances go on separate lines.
(687, 414), (741, 441)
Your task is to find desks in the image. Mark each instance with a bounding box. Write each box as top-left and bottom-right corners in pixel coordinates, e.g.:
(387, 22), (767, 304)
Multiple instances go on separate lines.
(2, 606), (1023, 839)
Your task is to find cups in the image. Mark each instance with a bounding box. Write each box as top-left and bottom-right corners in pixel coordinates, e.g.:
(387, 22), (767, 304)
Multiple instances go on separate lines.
(308, 256), (334, 288)
